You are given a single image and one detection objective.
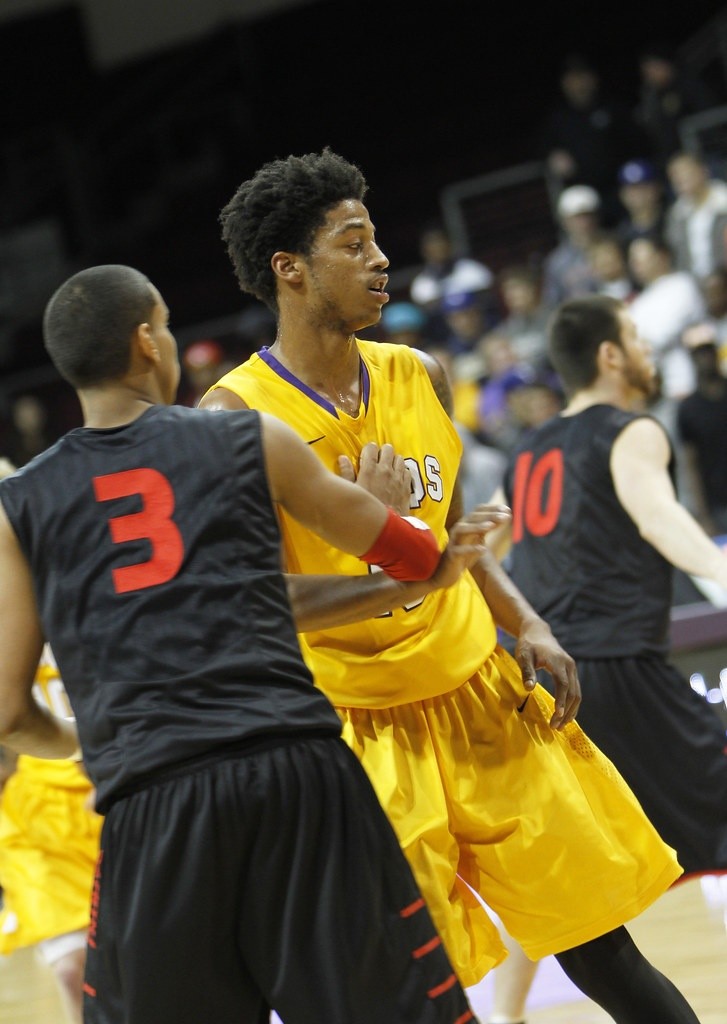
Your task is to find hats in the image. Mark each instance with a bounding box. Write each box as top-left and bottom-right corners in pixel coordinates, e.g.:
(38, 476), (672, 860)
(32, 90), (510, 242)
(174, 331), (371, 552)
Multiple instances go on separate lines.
(684, 323), (716, 353)
(500, 355), (565, 401)
(617, 159), (659, 184)
(381, 300), (425, 335)
(440, 291), (476, 311)
(557, 184), (599, 217)
(182, 341), (223, 367)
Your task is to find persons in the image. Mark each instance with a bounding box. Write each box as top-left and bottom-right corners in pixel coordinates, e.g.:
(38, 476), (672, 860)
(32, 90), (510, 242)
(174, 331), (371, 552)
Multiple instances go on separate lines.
(0, 141), (727, 1024)
